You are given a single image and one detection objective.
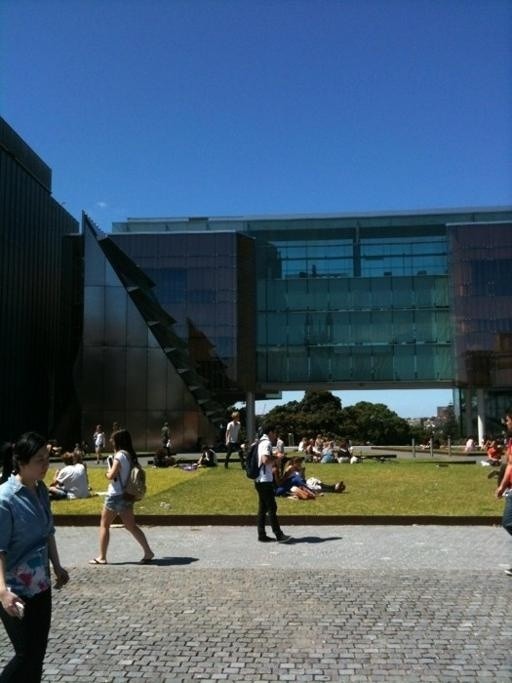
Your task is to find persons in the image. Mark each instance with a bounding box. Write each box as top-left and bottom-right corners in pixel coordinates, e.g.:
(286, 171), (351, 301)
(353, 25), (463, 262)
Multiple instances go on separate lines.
(161, 422), (171, 458)
(93, 425), (106, 464)
(0, 431), (69, 683)
(273, 433), (353, 500)
(419, 409), (512, 575)
(224, 412), (247, 470)
(88, 429), (154, 564)
(253, 421), (293, 543)
(45, 439), (91, 499)
(197, 445), (217, 468)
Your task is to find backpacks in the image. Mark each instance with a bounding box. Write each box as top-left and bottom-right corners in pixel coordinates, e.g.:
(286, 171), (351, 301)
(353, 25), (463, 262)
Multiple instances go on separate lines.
(117, 450), (146, 501)
(246, 439), (270, 479)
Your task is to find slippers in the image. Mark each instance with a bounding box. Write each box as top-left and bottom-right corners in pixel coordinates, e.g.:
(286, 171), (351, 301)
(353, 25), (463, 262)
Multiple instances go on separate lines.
(89, 559), (107, 564)
(140, 554), (154, 564)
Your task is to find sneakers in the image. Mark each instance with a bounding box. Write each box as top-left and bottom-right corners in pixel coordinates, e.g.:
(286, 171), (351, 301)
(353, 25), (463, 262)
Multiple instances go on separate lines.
(259, 536), (293, 543)
(335, 481), (345, 493)
